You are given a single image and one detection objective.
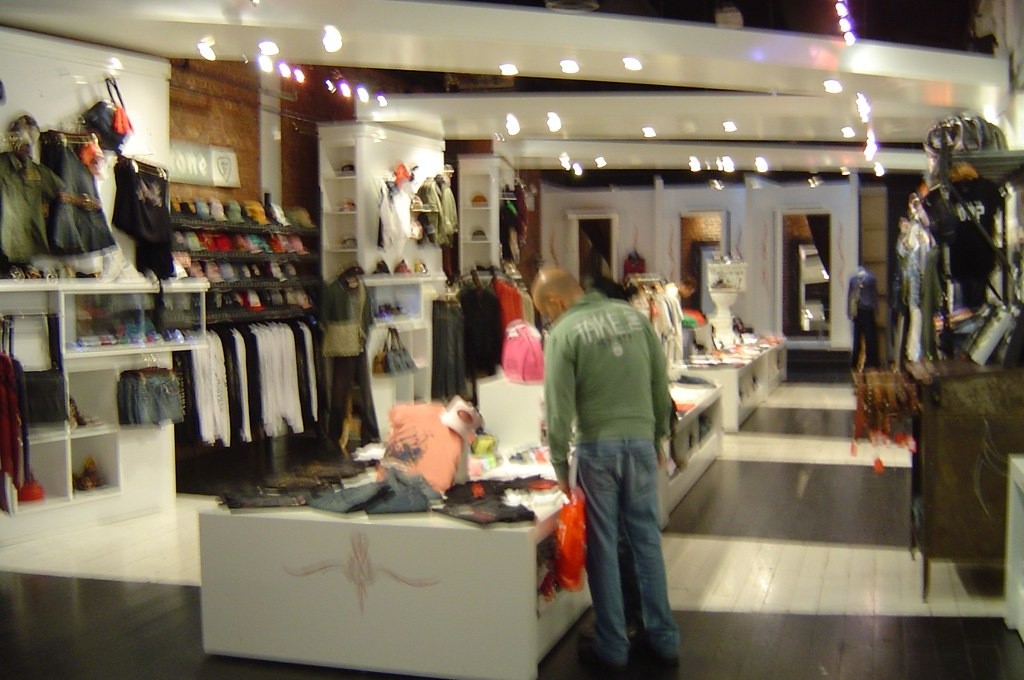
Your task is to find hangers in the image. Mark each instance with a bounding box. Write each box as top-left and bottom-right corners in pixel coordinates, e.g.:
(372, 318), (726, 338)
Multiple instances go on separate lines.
(629, 277), (667, 298)
(127, 155), (166, 180)
(4, 132), (22, 157)
(57, 120), (98, 146)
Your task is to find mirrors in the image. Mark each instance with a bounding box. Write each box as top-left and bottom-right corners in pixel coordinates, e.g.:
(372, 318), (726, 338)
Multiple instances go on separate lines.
(574, 213), (615, 286)
(777, 208), (832, 342)
(676, 207), (730, 312)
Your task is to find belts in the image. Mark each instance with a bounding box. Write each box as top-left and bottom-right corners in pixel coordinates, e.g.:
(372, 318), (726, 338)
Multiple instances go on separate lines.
(854, 359), (933, 448)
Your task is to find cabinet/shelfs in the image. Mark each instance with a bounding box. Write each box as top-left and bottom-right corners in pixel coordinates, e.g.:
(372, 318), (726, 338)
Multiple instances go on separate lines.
(316, 120), (523, 430)
(202, 330), (788, 680)
(880, 147), (1023, 604)
(158, 212), (319, 325)
(0, 277), (211, 544)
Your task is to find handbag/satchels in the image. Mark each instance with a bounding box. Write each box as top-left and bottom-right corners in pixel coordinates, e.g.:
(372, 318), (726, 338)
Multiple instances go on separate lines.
(81, 76), (131, 150)
(372, 328), (417, 376)
(502, 319), (544, 384)
(555, 487), (589, 590)
(322, 322), (366, 357)
(5, 370), (71, 423)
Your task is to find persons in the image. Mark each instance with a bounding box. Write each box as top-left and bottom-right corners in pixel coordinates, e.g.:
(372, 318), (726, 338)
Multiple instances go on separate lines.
(319, 262), (381, 459)
(847, 266), (883, 375)
(528, 265), (685, 679)
(648, 274), (700, 386)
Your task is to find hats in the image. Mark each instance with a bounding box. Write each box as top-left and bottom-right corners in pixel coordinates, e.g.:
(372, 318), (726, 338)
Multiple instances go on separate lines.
(342, 234), (357, 248)
(172, 197), (317, 307)
(338, 164), (354, 177)
(339, 201), (356, 213)
(337, 267), (364, 279)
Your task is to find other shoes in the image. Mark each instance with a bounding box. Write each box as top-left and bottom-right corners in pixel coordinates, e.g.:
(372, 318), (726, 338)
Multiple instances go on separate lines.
(579, 622), (636, 640)
(577, 650), (620, 680)
(630, 631), (681, 670)
